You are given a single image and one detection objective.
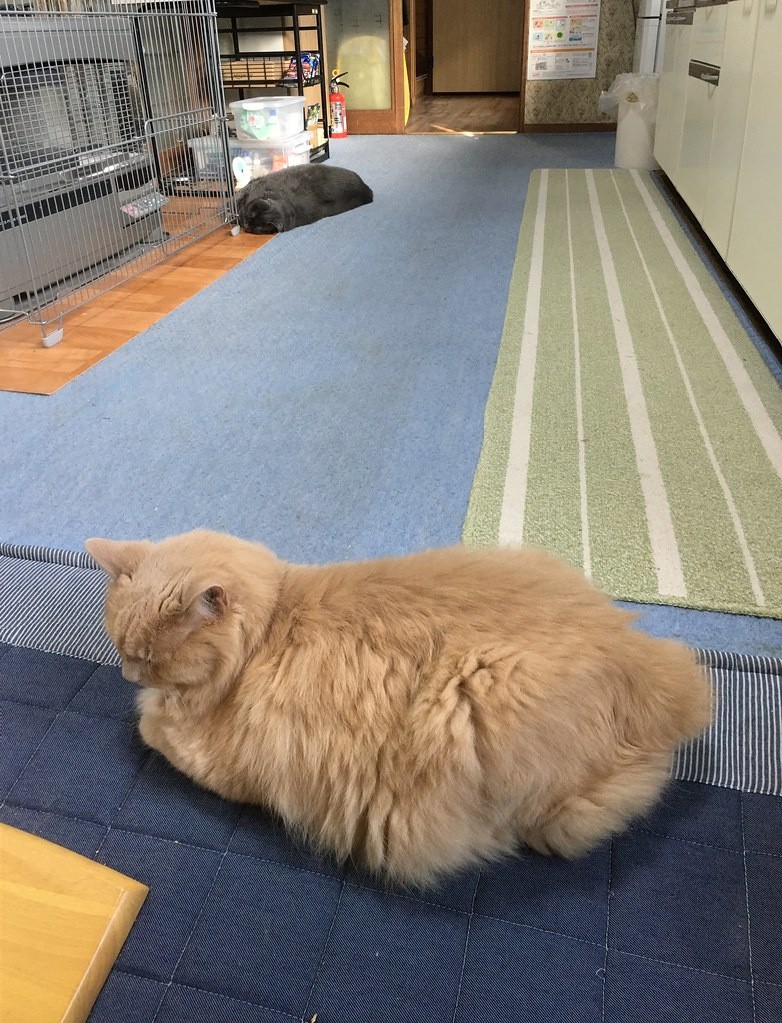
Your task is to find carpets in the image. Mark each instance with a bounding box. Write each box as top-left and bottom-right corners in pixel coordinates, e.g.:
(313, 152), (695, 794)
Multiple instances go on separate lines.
(464, 167), (782, 619)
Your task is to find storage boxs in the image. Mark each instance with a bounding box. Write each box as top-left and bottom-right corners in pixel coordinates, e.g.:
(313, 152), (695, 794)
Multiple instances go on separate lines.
(188, 96), (329, 185)
(220, 59), (282, 89)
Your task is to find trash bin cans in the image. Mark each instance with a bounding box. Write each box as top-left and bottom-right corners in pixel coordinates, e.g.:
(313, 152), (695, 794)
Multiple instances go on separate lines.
(615, 73), (661, 170)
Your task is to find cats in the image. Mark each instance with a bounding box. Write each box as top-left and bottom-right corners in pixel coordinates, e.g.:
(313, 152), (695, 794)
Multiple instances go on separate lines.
(226, 164), (373, 235)
(81, 529), (712, 892)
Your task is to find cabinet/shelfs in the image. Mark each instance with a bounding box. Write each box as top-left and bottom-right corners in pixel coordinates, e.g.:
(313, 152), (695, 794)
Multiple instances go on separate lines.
(134, 0), (330, 196)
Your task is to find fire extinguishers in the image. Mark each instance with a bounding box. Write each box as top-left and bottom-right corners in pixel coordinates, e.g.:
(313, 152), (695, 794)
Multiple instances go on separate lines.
(329, 71), (350, 138)
